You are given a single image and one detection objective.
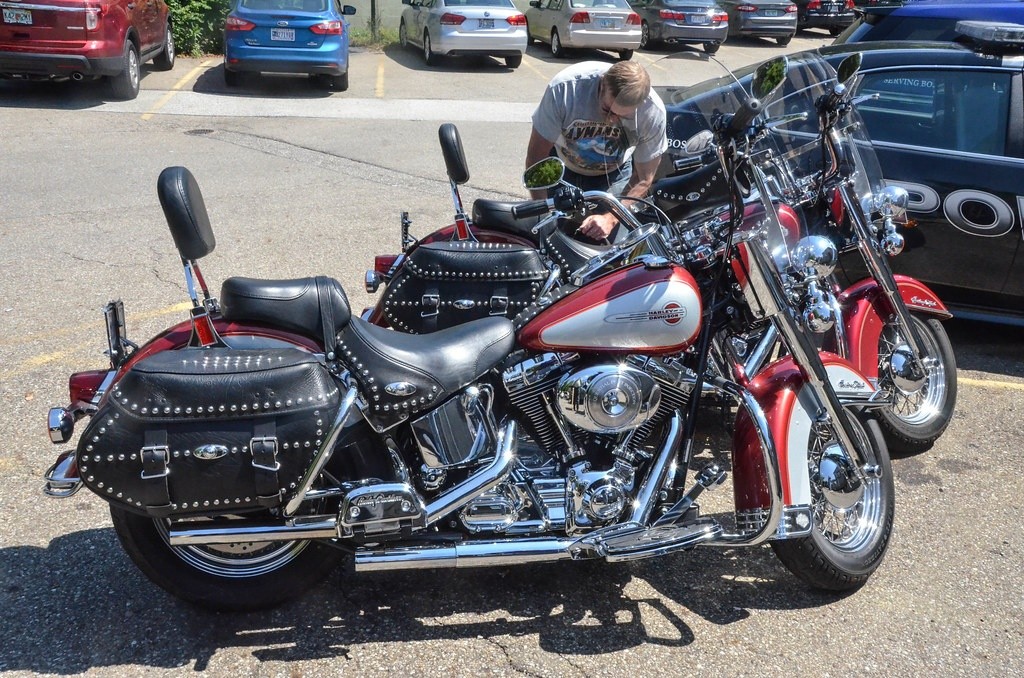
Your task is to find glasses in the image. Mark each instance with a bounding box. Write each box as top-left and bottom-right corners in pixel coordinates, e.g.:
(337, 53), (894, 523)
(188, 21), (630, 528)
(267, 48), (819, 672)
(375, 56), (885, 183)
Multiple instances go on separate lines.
(599, 88), (635, 121)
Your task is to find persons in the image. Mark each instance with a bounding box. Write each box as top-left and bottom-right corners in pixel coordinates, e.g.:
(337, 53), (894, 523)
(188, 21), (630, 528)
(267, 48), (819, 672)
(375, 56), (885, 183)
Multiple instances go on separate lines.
(525, 58), (671, 241)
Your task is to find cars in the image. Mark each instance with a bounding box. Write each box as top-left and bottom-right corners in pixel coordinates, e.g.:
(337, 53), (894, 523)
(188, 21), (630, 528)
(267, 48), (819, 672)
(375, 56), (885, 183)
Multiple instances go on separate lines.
(397, 0), (528, 69)
(642, 38), (1024, 337)
(627, 0), (730, 56)
(851, 0), (906, 23)
(222, 1), (356, 92)
(716, 0), (797, 45)
(523, 0), (643, 63)
(788, 0), (855, 39)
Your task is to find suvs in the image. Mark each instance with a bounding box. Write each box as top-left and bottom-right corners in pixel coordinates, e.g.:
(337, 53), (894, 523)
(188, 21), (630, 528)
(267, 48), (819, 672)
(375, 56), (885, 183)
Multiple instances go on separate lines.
(0, 0), (176, 101)
(829, 0), (1024, 49)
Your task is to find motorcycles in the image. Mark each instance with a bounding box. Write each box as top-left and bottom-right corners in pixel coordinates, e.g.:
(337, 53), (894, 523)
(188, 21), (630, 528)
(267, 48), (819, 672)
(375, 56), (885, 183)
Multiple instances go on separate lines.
(359, 36), (961, 457)
(42, 53), (896, 616)
